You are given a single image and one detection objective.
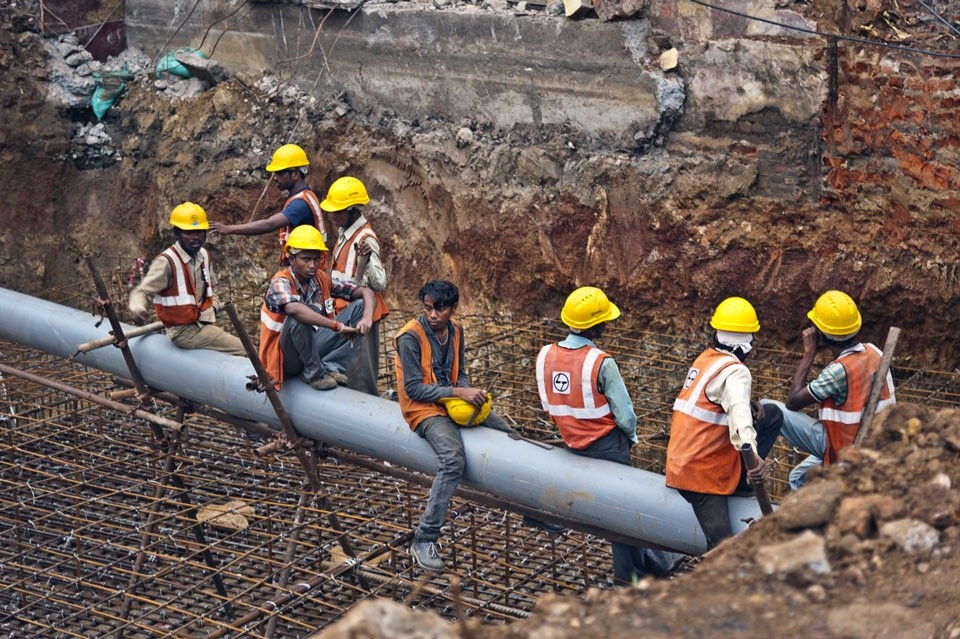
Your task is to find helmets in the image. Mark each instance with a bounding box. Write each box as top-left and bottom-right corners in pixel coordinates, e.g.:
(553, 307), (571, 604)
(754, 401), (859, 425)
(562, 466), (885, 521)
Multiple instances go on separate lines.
(265, 144), (309, 171)
(807, 291), (862, 335)
(320, 176), (369, 212)
(438, 393), (492, 425)
(286, 225), (329, 250)
(169, 202), (209, 230)
(561, 287), (620, 329)
(710, 297), (760, 332)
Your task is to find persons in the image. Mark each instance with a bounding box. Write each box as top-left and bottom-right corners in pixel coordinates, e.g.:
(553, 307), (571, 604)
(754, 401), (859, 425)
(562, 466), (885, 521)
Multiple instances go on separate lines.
(258, 224), (378, 390)
(536, 286), (675, 583)
(320, 177), (390, 396)
(208, 144), (328, 271)
(761, 290), (896, 490)
(392, 279), (566, 571)
(666, 297), (784, 552)
(127, 203), (260, 440)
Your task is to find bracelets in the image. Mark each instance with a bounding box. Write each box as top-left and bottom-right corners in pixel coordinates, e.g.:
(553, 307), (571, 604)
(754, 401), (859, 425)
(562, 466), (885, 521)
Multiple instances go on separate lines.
(333, 320), (344, 333)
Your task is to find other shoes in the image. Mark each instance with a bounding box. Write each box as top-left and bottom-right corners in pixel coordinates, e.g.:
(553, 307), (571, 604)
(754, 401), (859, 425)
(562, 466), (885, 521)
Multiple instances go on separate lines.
(410, 538), (445, 571)
(731, 487), (754, 497)
(523, 515), (566, 534)
(327, 372), (348, 384)
(310, 374), (337, 390)
